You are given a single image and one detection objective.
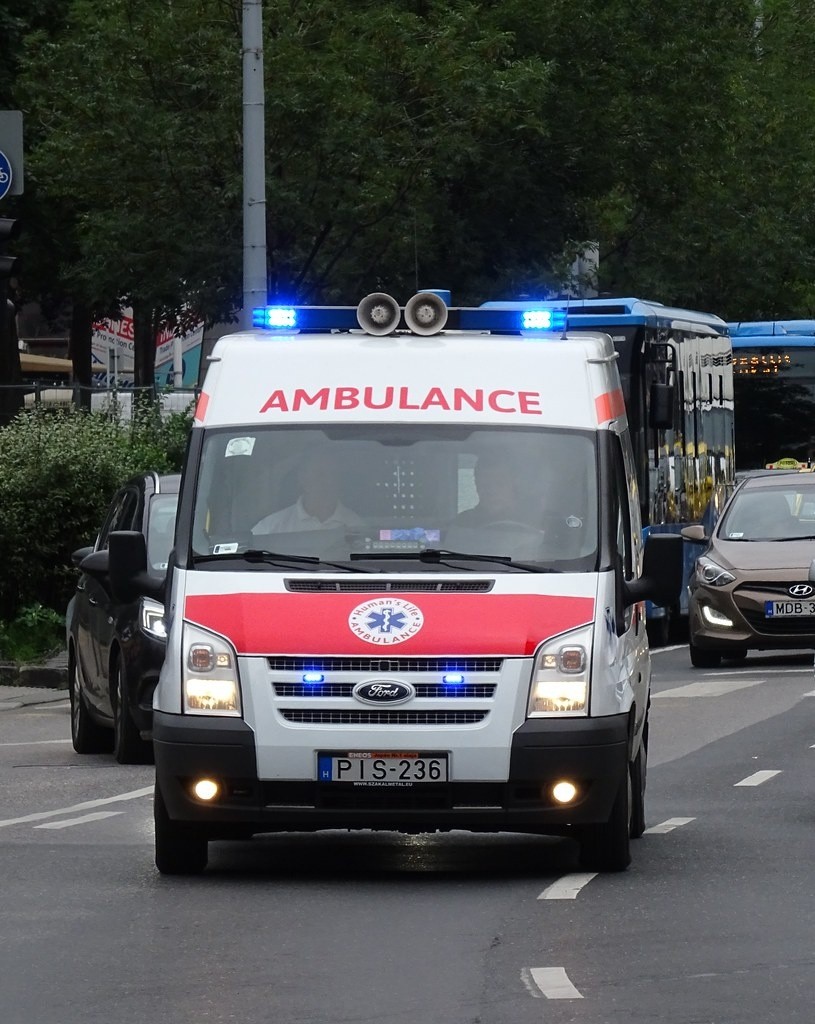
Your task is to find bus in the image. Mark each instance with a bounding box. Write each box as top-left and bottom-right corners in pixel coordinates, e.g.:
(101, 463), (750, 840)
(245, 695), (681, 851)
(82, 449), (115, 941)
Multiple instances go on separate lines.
(726, 319), (815, 473)
(477, 297), (735, 647)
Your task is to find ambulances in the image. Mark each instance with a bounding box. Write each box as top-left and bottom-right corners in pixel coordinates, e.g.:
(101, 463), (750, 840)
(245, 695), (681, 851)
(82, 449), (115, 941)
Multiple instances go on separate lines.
(150, 288), (683, 877)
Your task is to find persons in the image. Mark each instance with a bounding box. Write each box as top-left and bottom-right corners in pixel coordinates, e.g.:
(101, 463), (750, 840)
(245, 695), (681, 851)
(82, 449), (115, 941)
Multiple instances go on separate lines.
(447, 448), (556, 563)
(248, 457), (367, 558)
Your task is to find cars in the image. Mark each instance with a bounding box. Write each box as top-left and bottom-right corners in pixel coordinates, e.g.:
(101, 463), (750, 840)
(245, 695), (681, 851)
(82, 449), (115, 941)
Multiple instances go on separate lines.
(733, 456), (814, 522)
(679, 473), (815, 670)
(65, 473), (185, 767)
